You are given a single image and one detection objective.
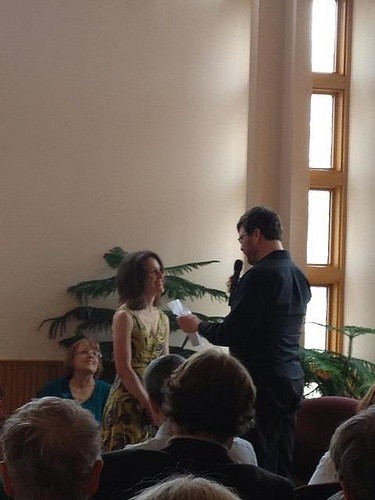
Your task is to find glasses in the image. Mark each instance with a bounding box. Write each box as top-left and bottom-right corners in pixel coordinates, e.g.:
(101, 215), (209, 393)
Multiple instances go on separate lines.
(75, 349), (102, 358)
(238, 234), (249, 244)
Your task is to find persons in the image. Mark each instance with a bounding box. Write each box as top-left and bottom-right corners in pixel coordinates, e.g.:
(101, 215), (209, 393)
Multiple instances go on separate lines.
(99, 248), (171, 452)
(125, 354), (259, 467)
(130, 475), (240, 500)
(90, 348), (296, 500)
(0, 396), (104, 500)
(328, 405), (375, 500)
(34, 337), (114, 432)
(307, 378), (375, 488)
(176, 205), (311, 488)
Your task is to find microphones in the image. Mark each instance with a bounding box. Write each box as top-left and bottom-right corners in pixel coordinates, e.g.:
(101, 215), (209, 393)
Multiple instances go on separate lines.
(228, 259), (243, 306)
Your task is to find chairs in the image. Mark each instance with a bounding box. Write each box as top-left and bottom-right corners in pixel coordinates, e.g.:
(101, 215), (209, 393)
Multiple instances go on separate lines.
(293, 395), (361, 482)
